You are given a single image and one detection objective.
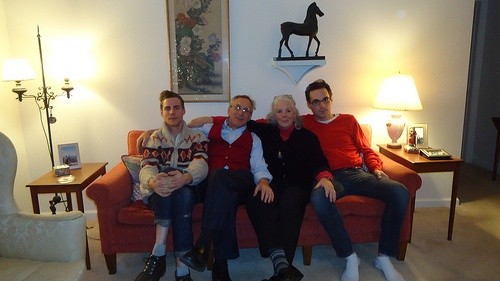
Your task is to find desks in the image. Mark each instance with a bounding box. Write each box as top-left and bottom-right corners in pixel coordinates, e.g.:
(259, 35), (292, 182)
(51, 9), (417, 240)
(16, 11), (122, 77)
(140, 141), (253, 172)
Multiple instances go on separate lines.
(25, 162), (108, 270)
(377, 143), (464, 244)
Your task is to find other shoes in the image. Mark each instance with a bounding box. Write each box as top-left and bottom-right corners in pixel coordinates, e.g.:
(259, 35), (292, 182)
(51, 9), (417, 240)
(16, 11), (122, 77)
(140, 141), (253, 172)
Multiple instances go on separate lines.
(178, 242), (209, 272)
(277, 263), (304, 281)
(175, 268), (193, 281)
(134, 253), (166, 281)
(261, 276), (278, 281)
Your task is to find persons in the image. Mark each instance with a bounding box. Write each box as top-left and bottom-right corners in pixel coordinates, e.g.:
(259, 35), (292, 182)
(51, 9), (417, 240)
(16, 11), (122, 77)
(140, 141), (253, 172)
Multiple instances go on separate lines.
(409, 127), (417, 145)
(134, 90), (210, 281)
(137, 79), (409, 281)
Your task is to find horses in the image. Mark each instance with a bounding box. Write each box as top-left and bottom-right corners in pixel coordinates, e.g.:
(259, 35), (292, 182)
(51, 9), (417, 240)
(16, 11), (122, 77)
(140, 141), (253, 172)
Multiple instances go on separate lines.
(278, 1), (324, 57)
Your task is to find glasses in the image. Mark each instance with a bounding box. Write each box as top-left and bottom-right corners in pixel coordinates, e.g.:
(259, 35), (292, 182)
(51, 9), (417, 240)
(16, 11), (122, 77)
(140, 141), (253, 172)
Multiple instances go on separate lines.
(230, 104), (253, 113)
(309, 96), (332, 106)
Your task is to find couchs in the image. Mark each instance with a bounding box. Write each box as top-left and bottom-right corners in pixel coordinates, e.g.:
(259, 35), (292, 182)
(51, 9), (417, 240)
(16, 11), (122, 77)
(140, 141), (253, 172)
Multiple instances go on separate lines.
(0, 132), (87, 281)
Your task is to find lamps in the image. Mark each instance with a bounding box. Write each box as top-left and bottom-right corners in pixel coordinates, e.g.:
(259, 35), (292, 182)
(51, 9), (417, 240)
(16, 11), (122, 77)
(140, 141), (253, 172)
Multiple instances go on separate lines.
(1, 22), (95, 214)
(372, 75), (424, 148)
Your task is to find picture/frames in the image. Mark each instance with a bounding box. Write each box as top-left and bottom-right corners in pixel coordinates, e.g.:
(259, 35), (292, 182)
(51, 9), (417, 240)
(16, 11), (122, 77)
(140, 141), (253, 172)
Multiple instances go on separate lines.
(57, 142), (81, 169)
(407, 124), (428, 149)
(165, 0), (231, 103)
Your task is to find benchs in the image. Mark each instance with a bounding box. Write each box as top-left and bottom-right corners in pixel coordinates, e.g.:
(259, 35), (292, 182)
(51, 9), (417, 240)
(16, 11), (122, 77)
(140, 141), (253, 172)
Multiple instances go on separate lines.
(85, 123), (423, 273)
